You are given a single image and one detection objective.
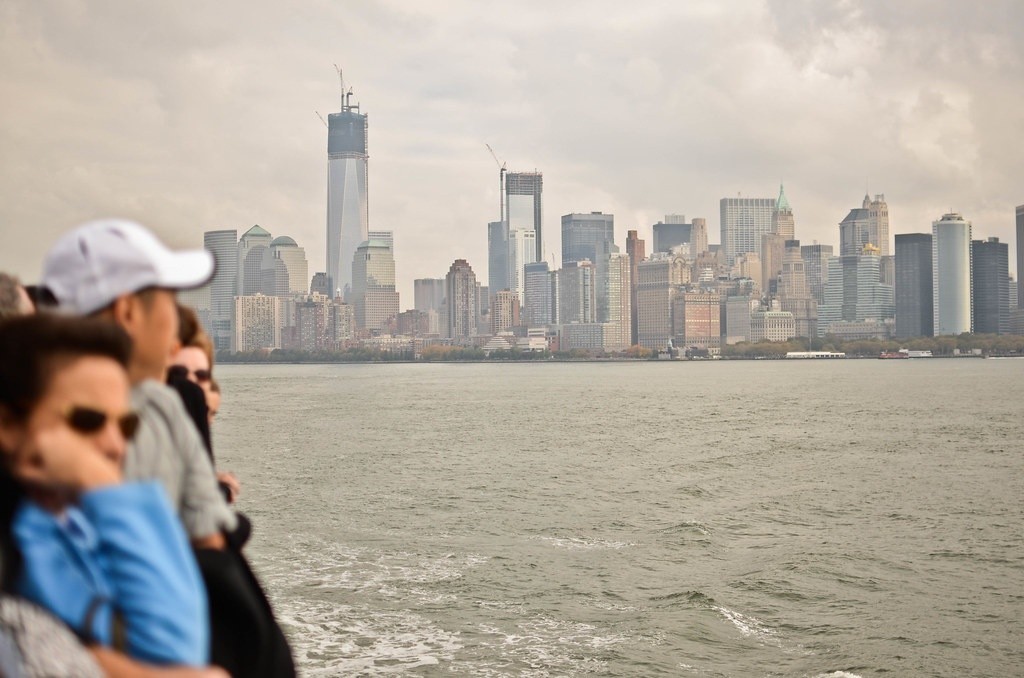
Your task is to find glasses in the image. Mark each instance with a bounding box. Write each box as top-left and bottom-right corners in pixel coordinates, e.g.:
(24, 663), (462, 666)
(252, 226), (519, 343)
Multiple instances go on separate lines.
(163, 364), (211, 383)
(29, 405), (138, 440)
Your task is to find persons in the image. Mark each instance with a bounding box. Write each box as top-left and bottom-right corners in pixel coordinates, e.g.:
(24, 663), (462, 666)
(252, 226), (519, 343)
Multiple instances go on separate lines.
(0, 218), (299, 678)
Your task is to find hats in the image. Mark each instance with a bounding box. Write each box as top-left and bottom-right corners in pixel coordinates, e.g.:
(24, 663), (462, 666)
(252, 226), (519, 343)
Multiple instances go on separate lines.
(39, 218), (216, 324)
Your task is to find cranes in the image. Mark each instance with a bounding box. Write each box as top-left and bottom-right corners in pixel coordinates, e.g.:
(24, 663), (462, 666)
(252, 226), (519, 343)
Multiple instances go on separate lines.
(484, 142), (510, 222)
(333, 62), (348, 110)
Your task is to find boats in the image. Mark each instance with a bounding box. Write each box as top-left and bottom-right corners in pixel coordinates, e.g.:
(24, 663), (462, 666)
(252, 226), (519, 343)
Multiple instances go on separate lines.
(898, 348), (934, 358)
(878, 349), (910, 359)
(785, 351), (846, 359)
